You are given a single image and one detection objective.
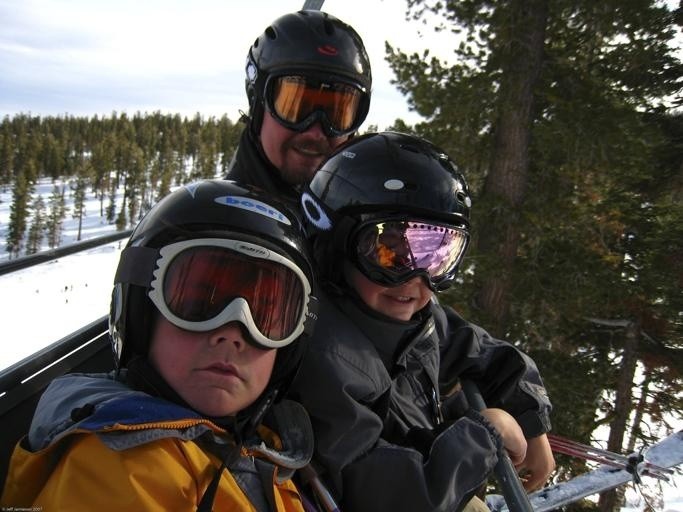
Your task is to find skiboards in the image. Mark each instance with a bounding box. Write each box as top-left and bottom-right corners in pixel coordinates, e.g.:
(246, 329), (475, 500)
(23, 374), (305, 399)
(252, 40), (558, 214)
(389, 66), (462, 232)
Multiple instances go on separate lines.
(490, 428), (681, 512)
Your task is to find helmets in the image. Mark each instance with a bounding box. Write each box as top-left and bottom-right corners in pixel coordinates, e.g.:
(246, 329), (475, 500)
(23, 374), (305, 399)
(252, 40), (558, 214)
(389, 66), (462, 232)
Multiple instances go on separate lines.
(109, 179), (313, 371)
(302, 131), (471, 241)
(245, 10), (372, 125)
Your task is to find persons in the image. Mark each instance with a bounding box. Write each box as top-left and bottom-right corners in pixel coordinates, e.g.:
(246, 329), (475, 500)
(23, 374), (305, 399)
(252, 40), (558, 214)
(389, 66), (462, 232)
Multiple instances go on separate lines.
(0, 177), (317, 512)
(223, 9), (372, 197)
(279, 132), (558, 510)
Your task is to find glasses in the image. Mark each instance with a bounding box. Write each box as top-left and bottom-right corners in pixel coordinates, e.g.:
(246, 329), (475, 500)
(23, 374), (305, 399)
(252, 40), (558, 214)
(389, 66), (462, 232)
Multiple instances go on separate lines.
(146, 235), (312, 347)
(266, 78), (368, 134)
(350, 217), (470, 294)
